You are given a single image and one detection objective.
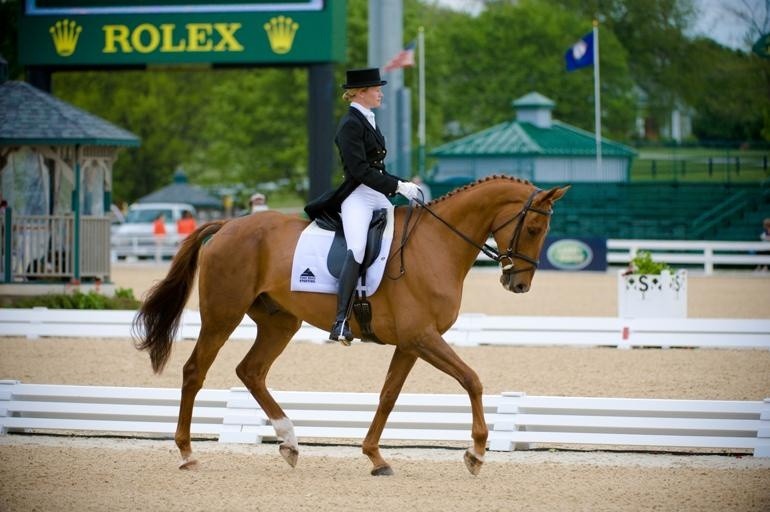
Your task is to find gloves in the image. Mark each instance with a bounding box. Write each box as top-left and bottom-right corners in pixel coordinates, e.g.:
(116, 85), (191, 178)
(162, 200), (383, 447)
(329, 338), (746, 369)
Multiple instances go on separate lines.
(395, 180), (421, 201)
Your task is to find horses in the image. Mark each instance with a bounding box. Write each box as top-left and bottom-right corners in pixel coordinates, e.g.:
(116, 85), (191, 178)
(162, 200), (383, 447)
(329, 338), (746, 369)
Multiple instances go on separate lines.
(131, 175), (572, 475)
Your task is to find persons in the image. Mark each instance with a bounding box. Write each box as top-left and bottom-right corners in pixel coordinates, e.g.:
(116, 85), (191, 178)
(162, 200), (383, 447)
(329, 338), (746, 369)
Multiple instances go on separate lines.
(177, 210), (197, 236)
(250, 192), (266, 214)
(310, 67), (416, 345)
(153, 212), (166, 236)
(754, 218), (770, 271)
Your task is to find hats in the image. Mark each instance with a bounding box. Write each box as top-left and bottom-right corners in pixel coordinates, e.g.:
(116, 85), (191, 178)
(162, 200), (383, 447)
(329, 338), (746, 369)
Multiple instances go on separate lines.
(342, 68), (387, 89)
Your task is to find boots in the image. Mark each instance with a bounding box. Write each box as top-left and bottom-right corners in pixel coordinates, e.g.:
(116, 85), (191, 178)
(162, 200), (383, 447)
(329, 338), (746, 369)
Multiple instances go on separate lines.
(329, 248), (363, 341)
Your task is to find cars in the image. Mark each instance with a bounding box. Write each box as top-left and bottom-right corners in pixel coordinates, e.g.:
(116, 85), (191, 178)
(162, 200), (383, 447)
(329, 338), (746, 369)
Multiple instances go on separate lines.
(114, 197), (196, 263)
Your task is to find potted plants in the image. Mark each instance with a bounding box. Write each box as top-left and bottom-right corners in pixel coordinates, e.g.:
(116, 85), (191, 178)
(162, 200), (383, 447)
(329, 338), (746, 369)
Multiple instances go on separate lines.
(617, 244), (689, 318)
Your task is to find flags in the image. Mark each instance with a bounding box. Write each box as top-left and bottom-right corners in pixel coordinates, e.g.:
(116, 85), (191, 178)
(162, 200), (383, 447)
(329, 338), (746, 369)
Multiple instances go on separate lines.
(385, 41), (415, 71)
(565, 31), (595, 72)
